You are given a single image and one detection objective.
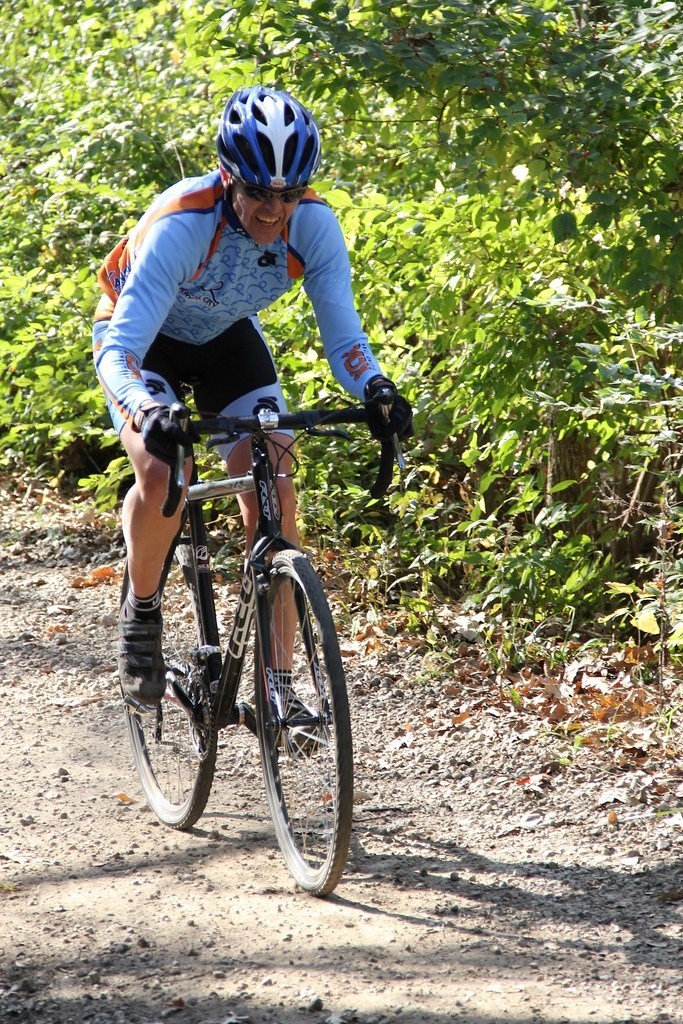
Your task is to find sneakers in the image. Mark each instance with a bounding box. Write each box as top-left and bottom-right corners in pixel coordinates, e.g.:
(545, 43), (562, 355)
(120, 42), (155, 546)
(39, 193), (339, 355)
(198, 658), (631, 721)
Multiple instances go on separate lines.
(118, 602), (167, 703)
(278, 695), (324, 750)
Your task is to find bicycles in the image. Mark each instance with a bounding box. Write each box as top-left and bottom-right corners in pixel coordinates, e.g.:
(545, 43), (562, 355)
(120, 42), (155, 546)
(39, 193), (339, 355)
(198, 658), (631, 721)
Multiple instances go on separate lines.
(107, 380), (410, 901)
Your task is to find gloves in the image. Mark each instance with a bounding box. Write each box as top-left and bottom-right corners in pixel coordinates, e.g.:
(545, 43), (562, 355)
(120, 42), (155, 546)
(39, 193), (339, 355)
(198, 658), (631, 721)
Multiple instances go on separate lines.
(142, 406), (203, 467)
(367, 377), (413, 441)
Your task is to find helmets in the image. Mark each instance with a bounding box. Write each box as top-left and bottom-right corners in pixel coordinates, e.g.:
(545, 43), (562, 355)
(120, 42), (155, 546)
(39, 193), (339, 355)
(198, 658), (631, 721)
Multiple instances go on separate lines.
(216, 85), (322, 189)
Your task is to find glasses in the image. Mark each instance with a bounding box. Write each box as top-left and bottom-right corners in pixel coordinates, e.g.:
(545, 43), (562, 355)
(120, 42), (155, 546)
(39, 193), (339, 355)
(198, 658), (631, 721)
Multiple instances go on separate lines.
(233, 176), (309, 202)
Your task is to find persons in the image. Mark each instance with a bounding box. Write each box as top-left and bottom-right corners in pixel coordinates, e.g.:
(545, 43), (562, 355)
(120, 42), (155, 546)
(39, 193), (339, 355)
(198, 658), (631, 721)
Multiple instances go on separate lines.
(92, 83), (413, 750)
